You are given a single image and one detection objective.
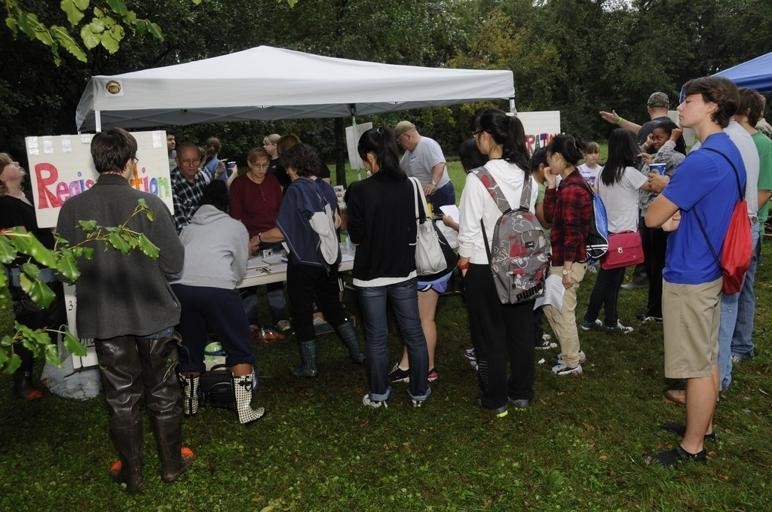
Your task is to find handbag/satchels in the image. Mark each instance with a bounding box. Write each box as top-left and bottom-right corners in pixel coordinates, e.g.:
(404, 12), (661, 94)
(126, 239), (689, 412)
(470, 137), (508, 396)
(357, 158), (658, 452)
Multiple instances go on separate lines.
(39, 326), (103, 403)
(599, 230), (646, 270)
(197, 364), (237, 410)
(307, 200), (340, 265)
(415, 216), (448, 277)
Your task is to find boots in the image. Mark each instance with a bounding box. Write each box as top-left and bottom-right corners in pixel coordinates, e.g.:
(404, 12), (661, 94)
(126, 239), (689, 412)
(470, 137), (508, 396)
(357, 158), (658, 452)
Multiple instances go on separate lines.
(107, 418), (145, 496)
(147, 411), (196, 484)
(232, 368), (266, 425)
(335, 322), (366, 369)
(11, 344), (43, 402)
(286, 338), (318, 379)
(178, 373), (199, 417)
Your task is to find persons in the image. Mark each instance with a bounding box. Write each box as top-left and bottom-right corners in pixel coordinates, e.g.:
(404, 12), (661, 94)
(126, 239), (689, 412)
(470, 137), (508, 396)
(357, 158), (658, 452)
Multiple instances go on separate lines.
(168, 108), (538, 429)
(0, 152), (53, 400)
(55, 126), (195, 494)
(527, 78), (772, 467)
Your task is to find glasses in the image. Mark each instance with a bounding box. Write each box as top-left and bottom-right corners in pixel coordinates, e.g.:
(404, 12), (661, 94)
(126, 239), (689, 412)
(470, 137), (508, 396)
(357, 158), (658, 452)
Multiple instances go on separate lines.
(471, 128), (488, 139)
(264, 142), (275, 147)
(178, 158), (202, 168)
(129, 156), (139, 165)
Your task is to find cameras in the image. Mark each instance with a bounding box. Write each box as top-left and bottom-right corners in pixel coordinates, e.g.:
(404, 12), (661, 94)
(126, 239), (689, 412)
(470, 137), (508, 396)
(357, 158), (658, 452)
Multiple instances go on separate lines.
(262, 248), (273, 258)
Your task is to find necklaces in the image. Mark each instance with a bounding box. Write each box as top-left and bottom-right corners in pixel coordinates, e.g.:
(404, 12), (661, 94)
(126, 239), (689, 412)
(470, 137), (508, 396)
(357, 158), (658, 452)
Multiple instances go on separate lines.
(2, 191), (26, 200)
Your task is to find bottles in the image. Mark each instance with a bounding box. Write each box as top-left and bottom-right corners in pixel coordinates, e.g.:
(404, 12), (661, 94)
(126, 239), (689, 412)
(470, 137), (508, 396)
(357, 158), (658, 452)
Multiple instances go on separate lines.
(227, 161), (237, 167)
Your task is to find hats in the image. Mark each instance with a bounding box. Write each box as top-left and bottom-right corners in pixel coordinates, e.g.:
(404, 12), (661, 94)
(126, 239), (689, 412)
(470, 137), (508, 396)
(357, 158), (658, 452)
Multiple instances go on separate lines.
(647, 91), (670, 108)
(394, 120), (416, 141)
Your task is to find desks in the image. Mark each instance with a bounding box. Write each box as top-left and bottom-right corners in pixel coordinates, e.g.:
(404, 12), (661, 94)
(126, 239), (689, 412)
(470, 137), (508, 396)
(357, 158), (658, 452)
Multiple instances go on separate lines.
(235, 239), (380, 327)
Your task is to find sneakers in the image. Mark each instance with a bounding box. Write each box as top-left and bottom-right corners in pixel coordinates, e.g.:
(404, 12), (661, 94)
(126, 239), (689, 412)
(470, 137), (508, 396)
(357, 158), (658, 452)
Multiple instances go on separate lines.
(406, 394), (423, 408)
(642, 442), (708, 471)
(664, 389), (687, 406)
(621, 280), (650, 290)
(604, 320), (634, 334)
(426, 370), (438, 382)
(636, 312), (664, 325)
(581, 319), (603, 332)
(554, 349), (587, 364)
(387, 363), (411, 383)
(362, 393), (388, 410)
(535, 331), (559, 350)
(475, 397), (509, 416)
(551, 362), (584, 377)
(507, 396), (531, 413)
(659, 422), (717, 445)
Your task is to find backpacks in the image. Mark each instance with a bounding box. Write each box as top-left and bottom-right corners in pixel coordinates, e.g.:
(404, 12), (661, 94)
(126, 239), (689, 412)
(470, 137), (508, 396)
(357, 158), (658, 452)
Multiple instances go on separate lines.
(719, 198), (755, 296)
(490, 206), (552, 306)
(586, 192), (611, 262)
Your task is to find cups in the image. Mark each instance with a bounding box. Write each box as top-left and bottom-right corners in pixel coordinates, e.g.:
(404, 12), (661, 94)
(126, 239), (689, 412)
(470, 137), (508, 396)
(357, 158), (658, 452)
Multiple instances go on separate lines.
(648, 163), (667, 176)
